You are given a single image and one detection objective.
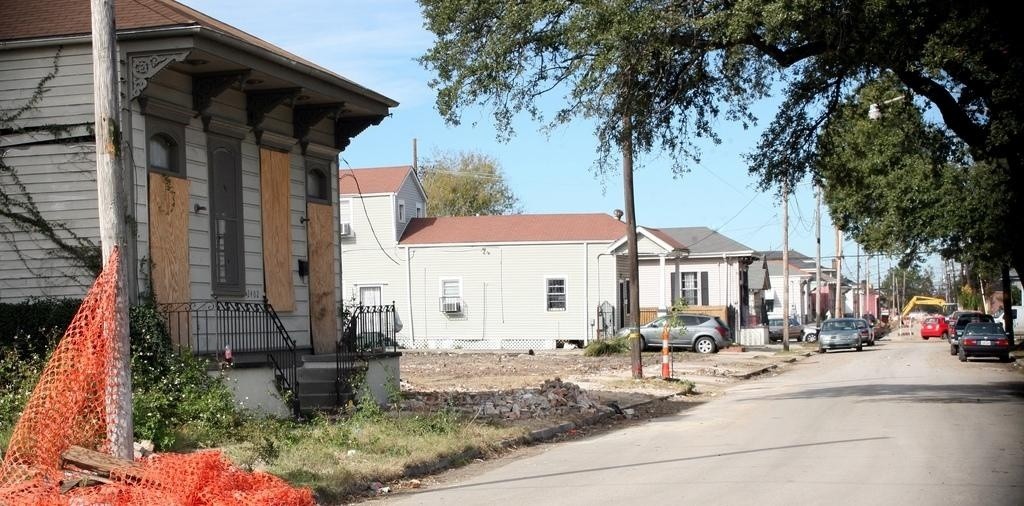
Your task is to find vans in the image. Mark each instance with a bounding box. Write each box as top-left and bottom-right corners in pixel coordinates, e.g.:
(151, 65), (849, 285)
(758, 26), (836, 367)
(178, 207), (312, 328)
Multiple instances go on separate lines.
(945, 310), (984, 344)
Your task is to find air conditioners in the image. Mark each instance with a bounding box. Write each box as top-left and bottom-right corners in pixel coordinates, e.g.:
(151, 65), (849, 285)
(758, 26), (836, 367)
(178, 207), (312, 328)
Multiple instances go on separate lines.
(443, 300), (460, 312)
(340, 222), (349, 235)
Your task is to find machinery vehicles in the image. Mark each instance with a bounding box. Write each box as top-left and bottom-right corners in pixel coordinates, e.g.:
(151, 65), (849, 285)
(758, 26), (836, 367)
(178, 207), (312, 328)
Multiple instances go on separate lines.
(896, 295), (957, 328)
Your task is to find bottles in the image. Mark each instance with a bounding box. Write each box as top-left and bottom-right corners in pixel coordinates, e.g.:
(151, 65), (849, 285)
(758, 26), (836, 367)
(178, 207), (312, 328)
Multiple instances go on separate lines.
(225, 343), (233, 365)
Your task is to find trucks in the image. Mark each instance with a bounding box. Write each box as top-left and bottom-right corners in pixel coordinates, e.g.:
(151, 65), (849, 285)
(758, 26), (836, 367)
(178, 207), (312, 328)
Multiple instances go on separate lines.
(992, 305), (1024, 336)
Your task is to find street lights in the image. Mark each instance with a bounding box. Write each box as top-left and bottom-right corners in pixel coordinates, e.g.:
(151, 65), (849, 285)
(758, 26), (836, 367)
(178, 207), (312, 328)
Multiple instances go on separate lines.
(866, 252), (875, 313)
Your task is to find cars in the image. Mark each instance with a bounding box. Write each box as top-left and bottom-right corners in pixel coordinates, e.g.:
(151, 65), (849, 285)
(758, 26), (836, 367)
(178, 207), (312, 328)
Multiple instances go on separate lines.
(921, 317), (949, 340)
(815, 317), (868, 354)
(768, 317), (805, 342)
(839, 313), (880, 340)
(956, 322), (1011, 362)
(857, 319), (875, 346)
(777, 324), (818, 344)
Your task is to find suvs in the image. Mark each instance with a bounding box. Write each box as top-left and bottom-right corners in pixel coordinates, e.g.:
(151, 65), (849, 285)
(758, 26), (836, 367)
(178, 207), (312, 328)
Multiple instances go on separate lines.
(949, 312), (995, 355)
(614, 312), (732, 354)
